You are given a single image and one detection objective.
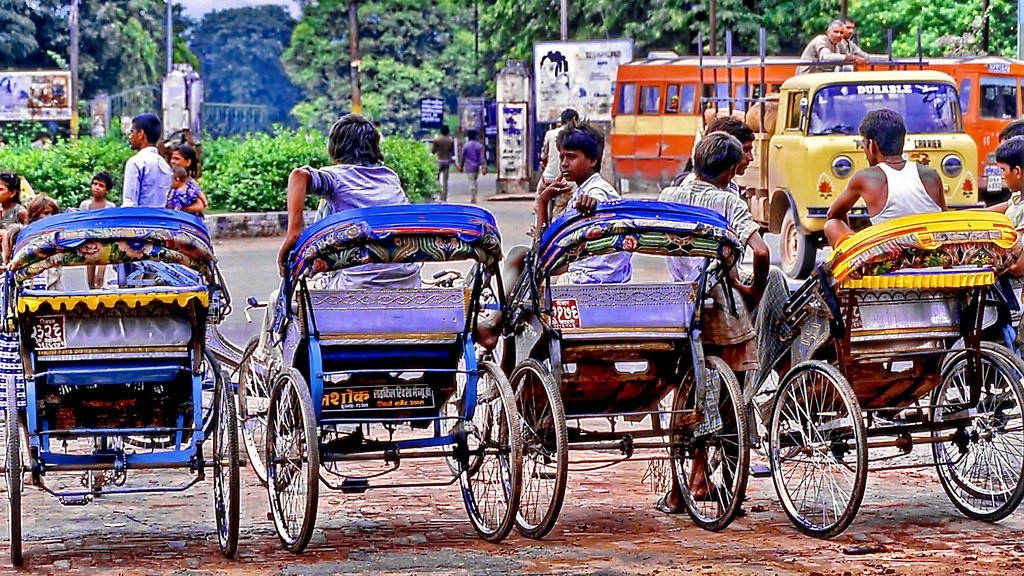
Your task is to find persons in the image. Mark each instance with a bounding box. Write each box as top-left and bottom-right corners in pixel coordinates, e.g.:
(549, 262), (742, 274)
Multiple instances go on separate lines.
(0, 173), (62, 492)
(654, 115), (802, 515)
(253, 113), (412, 370)
(371, 121), (385, 167)
(31, 133), (55, 150)
(78, 173), (116, 290)
(432, 124), (454, 204)
(652, 89), (716, 114)
(114, 114), (208, 290)
(0, 75), (67, 119)
(470, 121), (632, 351)
(534, 109), (579, 226)
(907, 104), (947, 133)
(957, 79), (1024, 413)
(794, 16), (868, 84)
(458, 130), (488, 205)
(824, 109), (948, 252)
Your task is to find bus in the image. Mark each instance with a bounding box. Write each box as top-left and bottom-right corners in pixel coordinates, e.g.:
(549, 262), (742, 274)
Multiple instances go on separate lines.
(611, 55), (1024, 203)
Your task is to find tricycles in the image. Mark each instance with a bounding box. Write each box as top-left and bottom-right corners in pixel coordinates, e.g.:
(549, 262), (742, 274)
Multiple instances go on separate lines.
(744, 209), (1024, 542)
(2, 202), (249, 568)
(432, 199), (749, 541)
(237, 202), (524, 555)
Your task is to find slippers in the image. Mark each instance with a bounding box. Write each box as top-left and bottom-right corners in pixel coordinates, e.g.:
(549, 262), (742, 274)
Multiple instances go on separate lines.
(654, 490), (686, 514)
(694, 484), (751, 501)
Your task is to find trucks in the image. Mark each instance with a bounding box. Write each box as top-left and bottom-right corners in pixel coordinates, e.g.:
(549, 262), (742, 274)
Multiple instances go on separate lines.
(691, 69), (981, 281)
